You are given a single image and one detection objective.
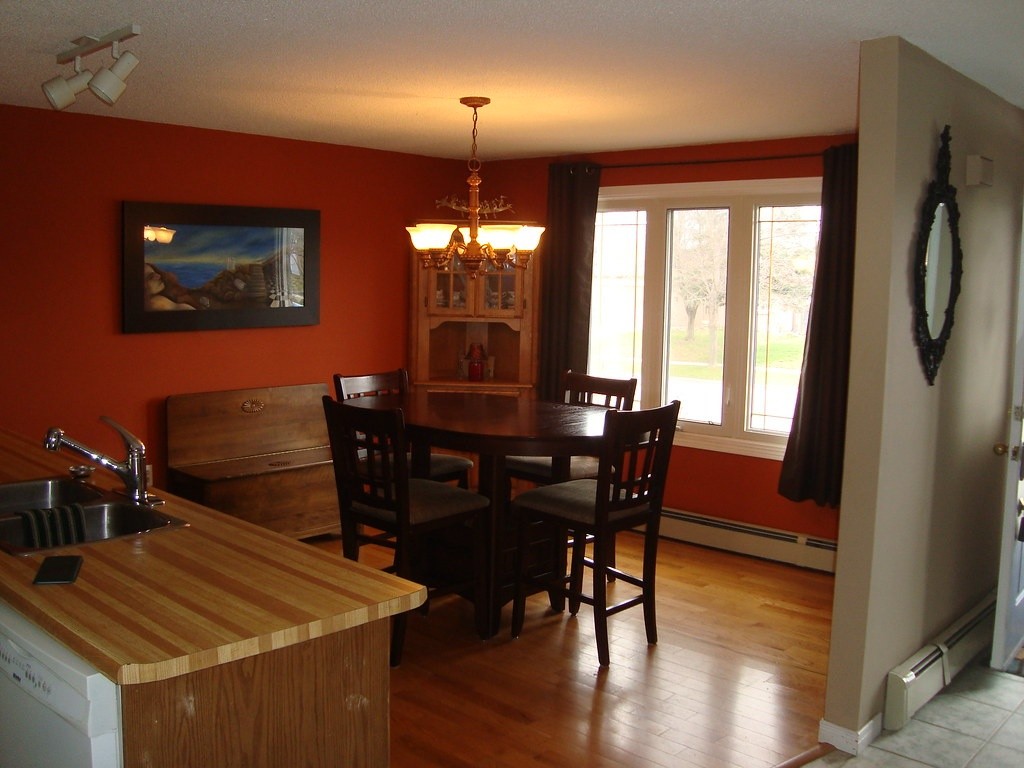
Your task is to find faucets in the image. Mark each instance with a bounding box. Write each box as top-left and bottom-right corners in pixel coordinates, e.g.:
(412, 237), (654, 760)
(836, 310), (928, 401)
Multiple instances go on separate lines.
(44, 415), (147, 500)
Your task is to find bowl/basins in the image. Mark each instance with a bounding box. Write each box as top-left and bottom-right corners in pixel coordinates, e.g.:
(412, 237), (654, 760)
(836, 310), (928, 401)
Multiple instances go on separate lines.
(437, 289), (515, 308)
(69, 464), (96, 483)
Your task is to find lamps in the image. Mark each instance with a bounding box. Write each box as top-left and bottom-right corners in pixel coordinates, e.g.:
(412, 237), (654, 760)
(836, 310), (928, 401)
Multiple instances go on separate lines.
(144, 225), (177, 248)
(405, 96), (546, 281)
(42, 55), (94, 112)
(86, 41), (140, 106)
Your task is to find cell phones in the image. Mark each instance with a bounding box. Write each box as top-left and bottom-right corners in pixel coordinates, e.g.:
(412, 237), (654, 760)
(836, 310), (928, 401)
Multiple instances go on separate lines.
(31, 555), (83, 585)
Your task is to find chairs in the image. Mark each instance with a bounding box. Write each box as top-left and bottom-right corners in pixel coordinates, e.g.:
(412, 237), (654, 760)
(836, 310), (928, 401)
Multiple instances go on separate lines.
(509, 400), (681, 667)
(323, 396), (492, 667)
(505, 369), (636, 610)
(334, 369), (474, 615)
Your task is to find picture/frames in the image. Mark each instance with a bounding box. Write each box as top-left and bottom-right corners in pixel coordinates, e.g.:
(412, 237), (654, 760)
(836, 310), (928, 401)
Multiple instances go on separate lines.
(119, 198), (321, 335)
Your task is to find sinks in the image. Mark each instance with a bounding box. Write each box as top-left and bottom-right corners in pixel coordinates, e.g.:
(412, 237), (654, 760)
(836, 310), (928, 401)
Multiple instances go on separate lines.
(0, 502), (190, 557)
(0, 476), (111, 514)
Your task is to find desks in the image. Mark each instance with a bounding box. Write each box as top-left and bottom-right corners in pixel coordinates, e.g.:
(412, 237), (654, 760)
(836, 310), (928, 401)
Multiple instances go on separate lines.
(342, 393), (651, 639)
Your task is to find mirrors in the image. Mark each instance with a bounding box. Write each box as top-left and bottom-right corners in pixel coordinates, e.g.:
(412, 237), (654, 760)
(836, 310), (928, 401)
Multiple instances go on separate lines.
(913, 124), (965, 386)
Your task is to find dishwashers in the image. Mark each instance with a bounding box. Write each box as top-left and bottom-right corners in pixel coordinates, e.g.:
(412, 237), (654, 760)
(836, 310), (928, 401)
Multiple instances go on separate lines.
(1, 598), (119, 768)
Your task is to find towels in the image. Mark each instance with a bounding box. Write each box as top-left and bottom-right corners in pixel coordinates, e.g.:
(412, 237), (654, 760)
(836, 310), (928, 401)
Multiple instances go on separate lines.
(14, 502), (87, 548)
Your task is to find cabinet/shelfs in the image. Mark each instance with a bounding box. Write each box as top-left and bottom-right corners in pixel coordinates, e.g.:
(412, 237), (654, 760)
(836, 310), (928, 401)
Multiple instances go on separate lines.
(410, 220), (541, 402)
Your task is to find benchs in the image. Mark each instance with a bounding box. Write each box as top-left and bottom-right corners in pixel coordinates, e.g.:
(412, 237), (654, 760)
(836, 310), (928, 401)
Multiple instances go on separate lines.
(165, 382), (342, 540)
